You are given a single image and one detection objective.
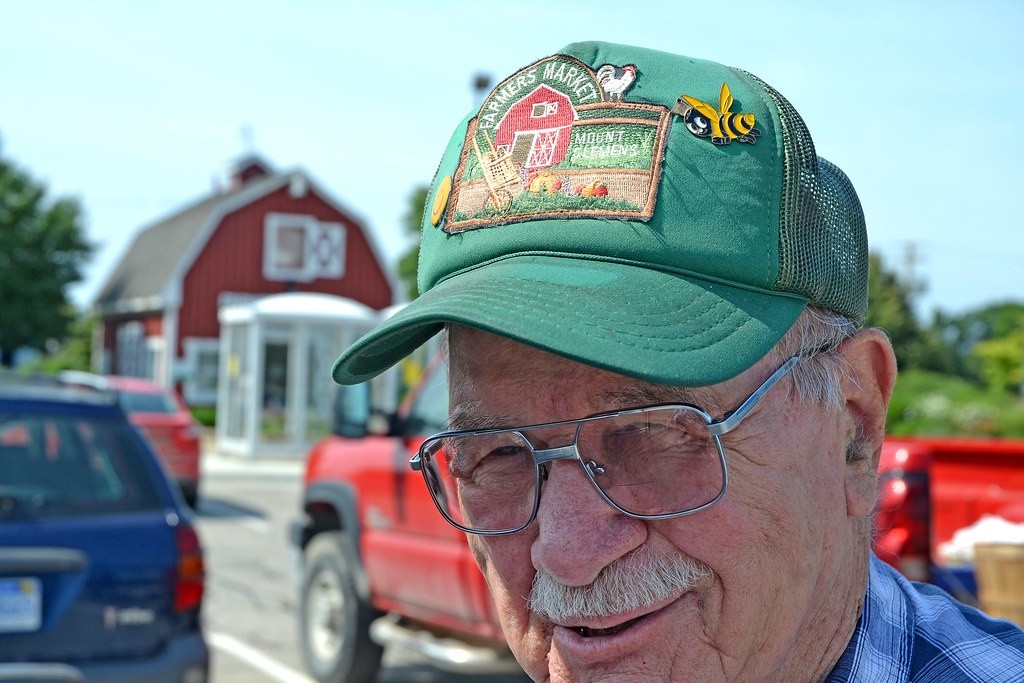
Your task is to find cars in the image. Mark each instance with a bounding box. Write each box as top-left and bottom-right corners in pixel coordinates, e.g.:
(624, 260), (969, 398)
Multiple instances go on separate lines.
(0, 377), (201, 507)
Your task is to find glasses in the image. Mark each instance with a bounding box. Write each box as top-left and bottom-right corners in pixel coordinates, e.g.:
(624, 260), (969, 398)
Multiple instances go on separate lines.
(410, 340), (842, 535)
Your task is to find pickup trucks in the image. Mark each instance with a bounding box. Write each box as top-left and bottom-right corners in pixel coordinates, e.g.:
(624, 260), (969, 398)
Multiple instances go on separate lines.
(288, 345), (1023, 683)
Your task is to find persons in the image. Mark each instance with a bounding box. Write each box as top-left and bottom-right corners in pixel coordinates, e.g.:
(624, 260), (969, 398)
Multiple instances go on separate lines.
(329, 38), (1024, 683)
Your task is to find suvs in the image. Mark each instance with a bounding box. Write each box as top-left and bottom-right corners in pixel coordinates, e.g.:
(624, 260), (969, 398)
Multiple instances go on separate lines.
(0, 368), (210, 683)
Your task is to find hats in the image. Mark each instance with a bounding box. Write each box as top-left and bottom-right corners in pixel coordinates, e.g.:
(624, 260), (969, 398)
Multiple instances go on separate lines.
(335, 41), (868, 387)
